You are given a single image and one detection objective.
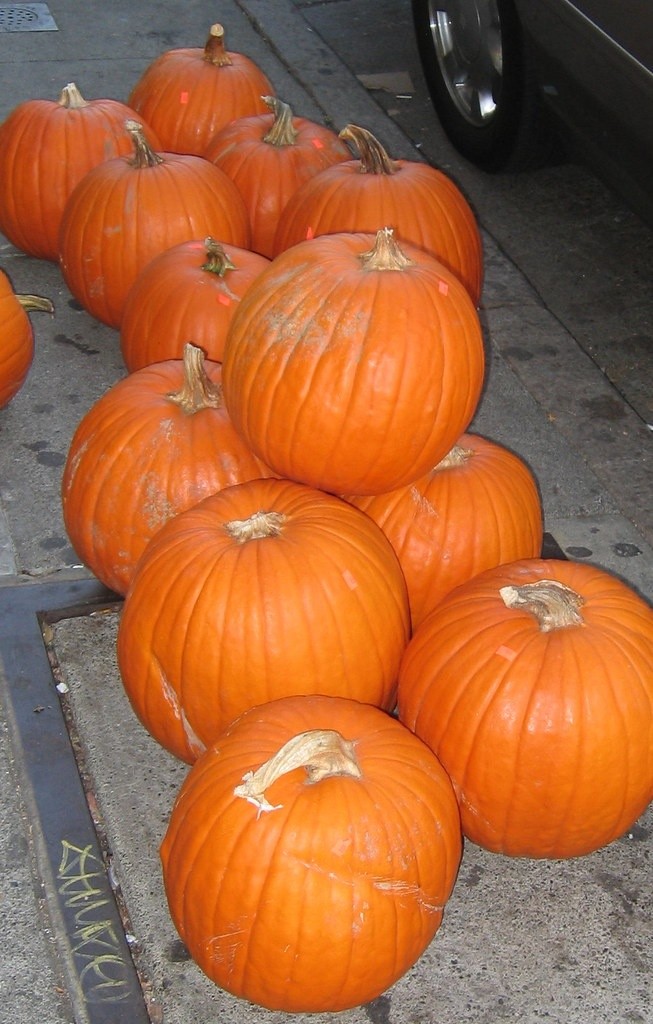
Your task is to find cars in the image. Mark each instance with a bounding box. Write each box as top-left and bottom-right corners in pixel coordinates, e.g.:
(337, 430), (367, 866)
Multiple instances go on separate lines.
(413, 1), (653, 228)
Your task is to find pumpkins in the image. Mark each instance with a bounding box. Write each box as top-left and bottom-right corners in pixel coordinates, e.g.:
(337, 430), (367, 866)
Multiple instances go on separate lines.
(221, 228), (485, 496)
(164, 694), (463, 1013)
(121, 241), (275, 374)
(127, 23), (275, 156)
(63, 343), (278, 605)
(274, 125), (484, 309)
(203, 97), (358, 246)
(0, 271), (55, 408)
(341, 432), (543, 633)
(61, 121), (250, 327)
(122, 479), (410, 767)
(0, 83), (160, 259)
(400, 558), (653, 860)
